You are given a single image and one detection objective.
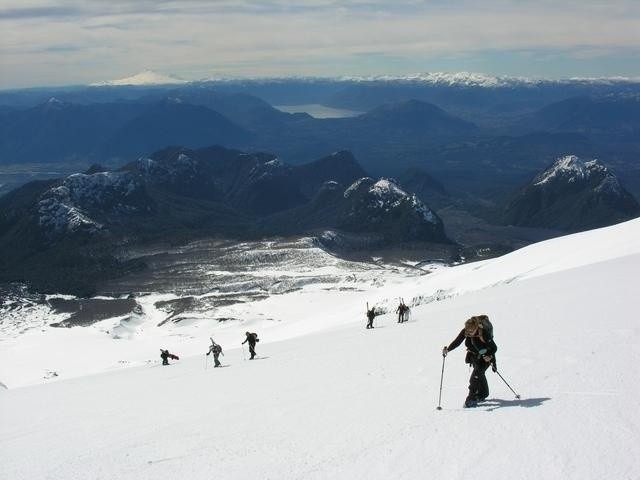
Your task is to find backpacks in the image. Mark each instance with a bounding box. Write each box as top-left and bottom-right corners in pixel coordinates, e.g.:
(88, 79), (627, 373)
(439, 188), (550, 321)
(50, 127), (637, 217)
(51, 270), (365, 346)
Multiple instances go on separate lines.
(465, 315), (493, 344)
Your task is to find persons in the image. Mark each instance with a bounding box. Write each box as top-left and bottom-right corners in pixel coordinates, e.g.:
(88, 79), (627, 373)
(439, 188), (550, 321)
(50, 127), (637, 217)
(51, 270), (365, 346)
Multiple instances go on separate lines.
(161, 350), (170, 365)
(206, 344), (222, 367)
(443, 318), (497, 407)
(402, 304), (411, 321)
(242, 332), (257, 360)
(366, 307), (376, 329)
(396, 303), (405, 323)
